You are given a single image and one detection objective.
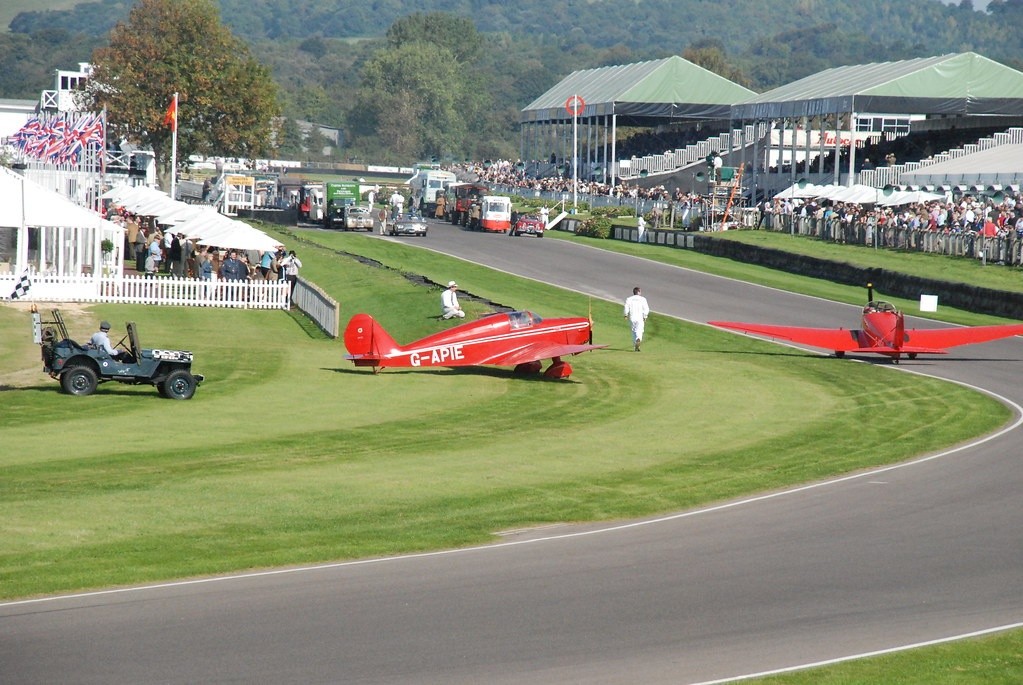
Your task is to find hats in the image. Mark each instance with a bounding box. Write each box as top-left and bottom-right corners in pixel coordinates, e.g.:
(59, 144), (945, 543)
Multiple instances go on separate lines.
(384, 205), (388, 208)
(448, 281), (458, 286)
(151, 252), (157, 254)
(289, 250), (295, 254)
(101, 321), (111, 330)
(139, 225), (146, 229)
(762, 194), (1021, 232)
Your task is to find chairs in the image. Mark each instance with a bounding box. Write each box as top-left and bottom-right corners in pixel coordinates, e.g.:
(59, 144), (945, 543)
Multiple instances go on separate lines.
(186, 257), (198, 278)
(91, 337), (97, 347)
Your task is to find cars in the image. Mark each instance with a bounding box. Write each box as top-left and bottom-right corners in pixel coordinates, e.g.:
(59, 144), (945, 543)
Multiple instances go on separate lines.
(388, 212), (431, 236)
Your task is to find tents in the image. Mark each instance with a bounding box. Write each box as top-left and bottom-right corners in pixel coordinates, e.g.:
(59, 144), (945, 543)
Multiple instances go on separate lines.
(520, 51), (1023, 197)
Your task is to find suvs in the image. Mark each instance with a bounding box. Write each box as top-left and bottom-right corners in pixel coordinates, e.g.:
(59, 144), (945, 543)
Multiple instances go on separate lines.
(27, 305), (208, 398)
(510, 208), (547, 238)
(344, 206), (376, 232)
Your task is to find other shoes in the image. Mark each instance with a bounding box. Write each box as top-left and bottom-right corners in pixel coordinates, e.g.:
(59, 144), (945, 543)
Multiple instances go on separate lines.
(438, 316), (444, 320)
(635, 347), (640, 351)
(635, 339), (640, 348)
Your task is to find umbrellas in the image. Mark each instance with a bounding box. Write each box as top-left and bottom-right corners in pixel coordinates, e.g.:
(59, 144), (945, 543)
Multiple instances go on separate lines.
(98, 184), (285, 252)
(772, 183), (946, 207)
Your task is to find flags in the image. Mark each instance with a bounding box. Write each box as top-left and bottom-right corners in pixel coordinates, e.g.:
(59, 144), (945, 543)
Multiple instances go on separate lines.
(4, 112), (104, 167)
(9, 268), (31, 299)
(162, 98), (176, 131)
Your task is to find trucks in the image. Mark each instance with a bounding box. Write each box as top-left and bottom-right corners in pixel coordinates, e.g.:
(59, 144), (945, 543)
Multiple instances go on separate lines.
(176, 168), (361, 229)
(444, 182), (490, 228)
(410, 169), (459, 217)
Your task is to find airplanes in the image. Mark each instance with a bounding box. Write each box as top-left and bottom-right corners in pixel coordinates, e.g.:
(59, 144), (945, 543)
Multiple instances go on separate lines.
(343, 298), (611, 383)
(704, 284), (1022, 362)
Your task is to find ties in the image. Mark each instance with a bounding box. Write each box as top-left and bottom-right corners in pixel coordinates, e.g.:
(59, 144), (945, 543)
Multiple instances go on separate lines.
(451, 293), (454, 305)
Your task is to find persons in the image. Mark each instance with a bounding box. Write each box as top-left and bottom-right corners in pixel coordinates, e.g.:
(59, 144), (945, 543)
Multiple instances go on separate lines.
(582, 119), (760, 162)
(438, 281), (465, 320)
(510, 315), (519, 329)
(713, 153), (722, 185)
(625, 287), (649, 351)
(368, 191), (374, 213)
(434, 194), (445, 217)
(509, 209), (519, 236)
(754, 194), (1023, 266)
(90, 321), (136, 364)
(185, 157), (274, 200)
(746, 125), (966, 172)
(863, 302), (876, 313)
(638, 215), (646, 243)
(102, 201), (302, 302)
(378, 190), (417, 236)
(441, 159), (711, 229)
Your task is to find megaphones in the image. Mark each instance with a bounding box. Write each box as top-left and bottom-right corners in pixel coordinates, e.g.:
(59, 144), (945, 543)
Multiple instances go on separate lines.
(875, 184), (893, 196)
(594, 166), (602, 175)
(517, 163), (525, 170)
(484, 160), (491, 167)
(693, 171), (706, 182)
(980, 190), (1004, 205)
(638, 169), (648, 178)
(789, 178), (807, 189)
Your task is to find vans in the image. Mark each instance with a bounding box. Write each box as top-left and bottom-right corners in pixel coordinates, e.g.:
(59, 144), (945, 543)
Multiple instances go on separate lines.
(480, 195), (512, 232)
